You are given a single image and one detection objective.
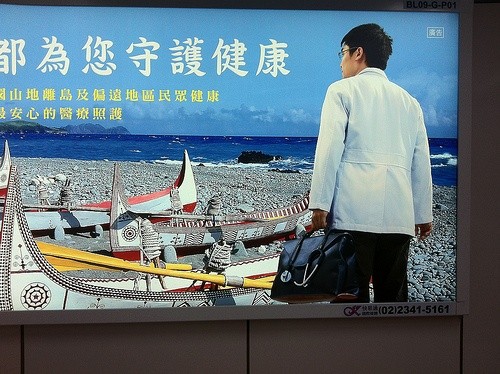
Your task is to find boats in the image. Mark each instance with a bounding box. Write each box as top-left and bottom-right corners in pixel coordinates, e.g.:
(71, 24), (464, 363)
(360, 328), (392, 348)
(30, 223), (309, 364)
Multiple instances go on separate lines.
(1, 139), (199, 230)
(109, 162), (314, 260)
(1, 163), (329, 311)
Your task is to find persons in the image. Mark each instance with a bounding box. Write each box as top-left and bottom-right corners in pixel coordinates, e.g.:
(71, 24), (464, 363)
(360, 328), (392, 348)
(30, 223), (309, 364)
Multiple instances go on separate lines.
(308, 22), (434, 302)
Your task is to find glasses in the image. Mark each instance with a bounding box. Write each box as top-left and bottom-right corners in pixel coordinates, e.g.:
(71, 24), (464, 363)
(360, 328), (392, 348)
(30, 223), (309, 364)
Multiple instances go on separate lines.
(337, 47), (359, 59)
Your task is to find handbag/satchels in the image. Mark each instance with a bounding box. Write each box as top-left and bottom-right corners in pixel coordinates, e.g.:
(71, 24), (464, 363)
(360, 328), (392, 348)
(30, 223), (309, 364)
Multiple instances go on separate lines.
(269, 218), (358, 302)
